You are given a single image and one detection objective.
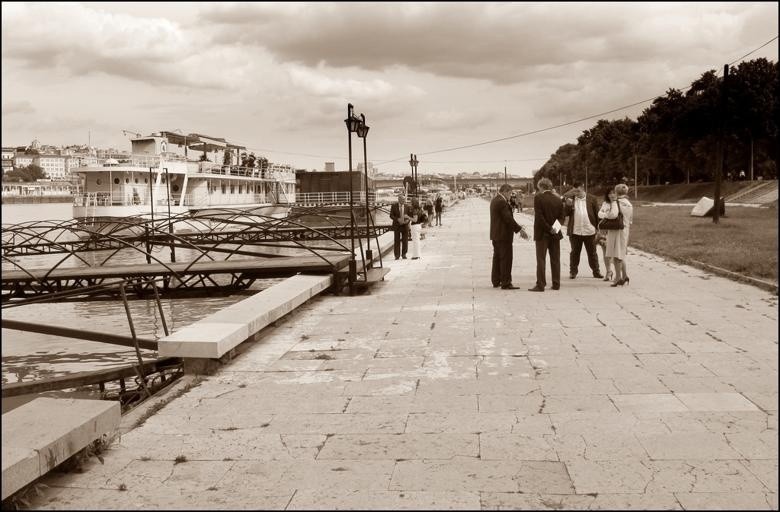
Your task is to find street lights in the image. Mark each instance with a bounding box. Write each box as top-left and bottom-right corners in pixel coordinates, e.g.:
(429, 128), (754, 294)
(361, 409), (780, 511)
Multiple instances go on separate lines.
(344, 103), (372, 295)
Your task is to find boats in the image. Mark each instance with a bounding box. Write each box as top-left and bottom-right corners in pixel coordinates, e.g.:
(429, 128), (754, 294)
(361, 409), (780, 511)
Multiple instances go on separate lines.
(71, 130), (297, 250)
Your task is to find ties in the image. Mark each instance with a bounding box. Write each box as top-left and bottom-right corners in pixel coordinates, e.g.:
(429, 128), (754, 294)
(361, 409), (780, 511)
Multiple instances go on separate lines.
(399, 205), (403, 221)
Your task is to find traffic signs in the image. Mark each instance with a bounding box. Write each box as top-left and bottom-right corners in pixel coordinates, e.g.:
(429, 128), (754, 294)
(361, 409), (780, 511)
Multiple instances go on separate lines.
(408, 154), (419, 205)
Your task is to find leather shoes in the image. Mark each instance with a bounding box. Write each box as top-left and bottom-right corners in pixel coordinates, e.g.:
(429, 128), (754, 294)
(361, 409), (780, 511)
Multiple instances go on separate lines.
(552, 285), (560, 289)
(495, 281), (519, 289)
(529, 286), (544, 291)
(395, 255), (407, 259)
(569, 272), (577, 278)
(594, 272), (603, 278)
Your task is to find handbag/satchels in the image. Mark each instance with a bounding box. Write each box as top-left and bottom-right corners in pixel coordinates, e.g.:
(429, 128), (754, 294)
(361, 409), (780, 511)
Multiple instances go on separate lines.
(598, 212), (624, 230)
(421, 214), (428, 223)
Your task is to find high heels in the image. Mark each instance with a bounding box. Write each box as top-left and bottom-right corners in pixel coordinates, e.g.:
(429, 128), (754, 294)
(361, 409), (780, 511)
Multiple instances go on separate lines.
(604, 270), (630, 286)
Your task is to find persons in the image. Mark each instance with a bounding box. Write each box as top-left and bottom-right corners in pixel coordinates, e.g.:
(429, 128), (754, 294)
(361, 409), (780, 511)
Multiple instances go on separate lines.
(223, 150), (268, 178)
(607, 183), (633, 287)
(739, 169), (745, 180)
(390, 191), (443, 260)
(486, 183), (529, 289)
(565, 182), (604, 278)
(726, 168), (738, 182)
(510, 191), (523, 213)
(597, 185), (615, 281)
(529, 177), (565, 291)
